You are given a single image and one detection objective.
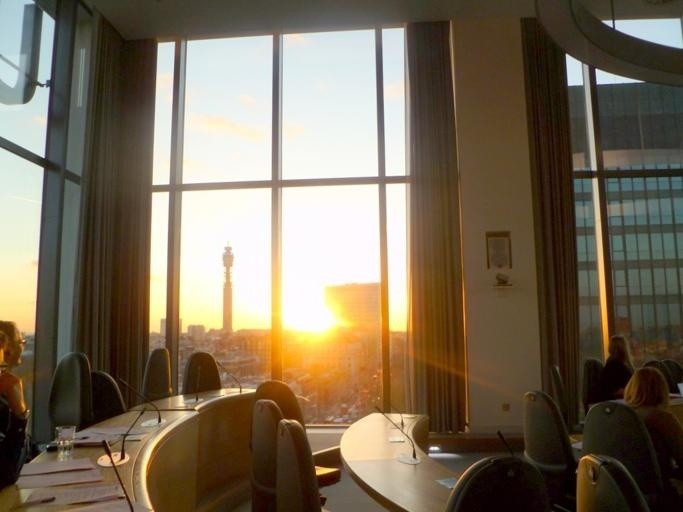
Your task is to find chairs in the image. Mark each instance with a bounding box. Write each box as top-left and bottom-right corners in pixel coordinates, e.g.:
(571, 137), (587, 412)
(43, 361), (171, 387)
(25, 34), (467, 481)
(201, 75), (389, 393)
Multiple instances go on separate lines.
(444, 358), (683, 512)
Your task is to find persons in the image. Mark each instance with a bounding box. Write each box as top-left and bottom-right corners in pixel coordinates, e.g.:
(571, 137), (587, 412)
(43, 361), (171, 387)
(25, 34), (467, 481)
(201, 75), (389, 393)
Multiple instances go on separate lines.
(622, 367), (683, 503)
(0, 321), (35, 489)
(603, 335), (632, 398)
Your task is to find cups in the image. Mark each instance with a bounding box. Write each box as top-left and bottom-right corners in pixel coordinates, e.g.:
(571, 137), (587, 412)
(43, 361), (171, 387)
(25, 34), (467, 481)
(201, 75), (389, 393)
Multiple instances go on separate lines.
(55, 425), (76, 456)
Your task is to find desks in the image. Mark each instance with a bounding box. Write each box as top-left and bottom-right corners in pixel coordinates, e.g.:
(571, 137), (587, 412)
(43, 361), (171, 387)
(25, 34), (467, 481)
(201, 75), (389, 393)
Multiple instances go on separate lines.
(0, 388), (258, 512)
(338, 413), (462, 512)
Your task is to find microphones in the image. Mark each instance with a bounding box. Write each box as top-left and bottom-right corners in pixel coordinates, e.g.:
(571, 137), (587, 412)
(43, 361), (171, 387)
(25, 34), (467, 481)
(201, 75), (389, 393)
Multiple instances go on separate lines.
(496, 430), (514, 456)
(369, 389), (403, 429)
(184, 366), (204, 404)
(119, 377), (166, 427)
(375, 406), (421, 464)
(97, 408), (147, 467)
(216, 361), (242, 393)
(101, 440), (134, 512)
